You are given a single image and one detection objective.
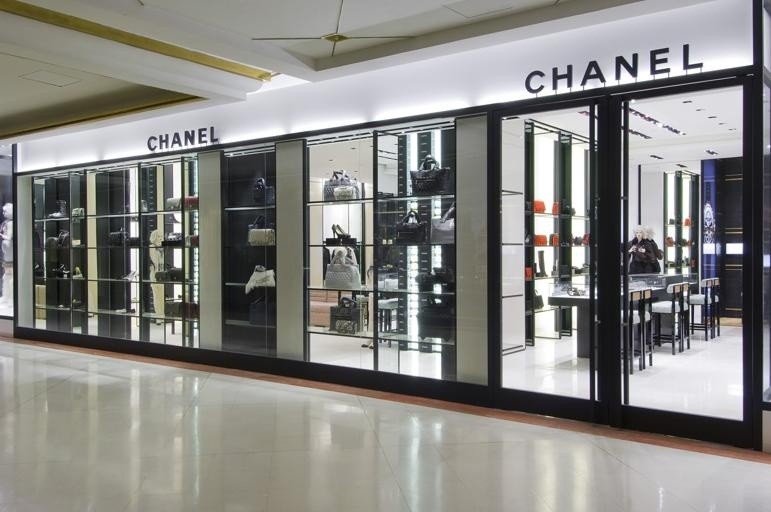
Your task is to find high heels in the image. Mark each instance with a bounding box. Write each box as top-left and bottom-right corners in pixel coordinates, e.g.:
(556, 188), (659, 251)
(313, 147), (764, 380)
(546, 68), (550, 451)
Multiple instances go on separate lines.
(332, 224), (345, 239)
(337, 225), (351, 238)
(73, 296), (81, 308)
(73, 265), (80, 276)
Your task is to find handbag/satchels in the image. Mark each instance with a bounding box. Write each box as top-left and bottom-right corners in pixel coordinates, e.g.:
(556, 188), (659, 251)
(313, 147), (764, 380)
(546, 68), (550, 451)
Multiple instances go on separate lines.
(325, 249), (362, 290)
(249, 177), (275, 207)
(243, 215), (276, 326)
(323, 169), (360, 201)
(395, 211), (426, 245)
(329, 297), (360, 334)
(163, 196), (199, 320)
(533, 291), (543, 309)
(410, 155), (454, 196)
(430, 207), (455, 245)
(418, 296), (452, 341)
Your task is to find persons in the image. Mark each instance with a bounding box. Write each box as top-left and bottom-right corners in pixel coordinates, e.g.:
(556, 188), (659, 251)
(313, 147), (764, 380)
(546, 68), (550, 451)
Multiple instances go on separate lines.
(704, 211), (715, 243)
(627, 225), (655, 274)
(0, 203), (13, 316)
(148, 229), (165, 325)
(644, 225), (664, 273)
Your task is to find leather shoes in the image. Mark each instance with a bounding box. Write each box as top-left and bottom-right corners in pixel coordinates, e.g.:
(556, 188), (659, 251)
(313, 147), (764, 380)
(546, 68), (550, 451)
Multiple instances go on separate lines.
(122, 271), (137, 282)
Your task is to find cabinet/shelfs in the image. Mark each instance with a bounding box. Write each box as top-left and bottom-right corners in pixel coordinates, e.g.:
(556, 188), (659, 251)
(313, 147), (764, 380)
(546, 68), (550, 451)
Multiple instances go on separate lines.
(221, 144), (276, 356)
(525, 122), (591, 345)
(309, 117), (455, 381)
(665, 166), (700, 287)
(15, 152), (200, 349)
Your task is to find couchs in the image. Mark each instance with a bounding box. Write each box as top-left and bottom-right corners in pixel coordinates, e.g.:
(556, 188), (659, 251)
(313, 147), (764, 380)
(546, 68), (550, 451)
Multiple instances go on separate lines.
(310, 288), (352, 327)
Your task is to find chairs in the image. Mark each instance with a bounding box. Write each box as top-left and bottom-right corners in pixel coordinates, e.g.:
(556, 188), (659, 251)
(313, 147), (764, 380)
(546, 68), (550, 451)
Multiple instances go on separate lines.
(615, 277), (721, 374)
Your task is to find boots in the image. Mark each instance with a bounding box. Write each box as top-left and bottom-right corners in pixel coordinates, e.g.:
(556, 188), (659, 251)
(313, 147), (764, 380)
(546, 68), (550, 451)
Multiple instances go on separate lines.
(48, 199), (67, 218)
(538, 250), (547, 278)
